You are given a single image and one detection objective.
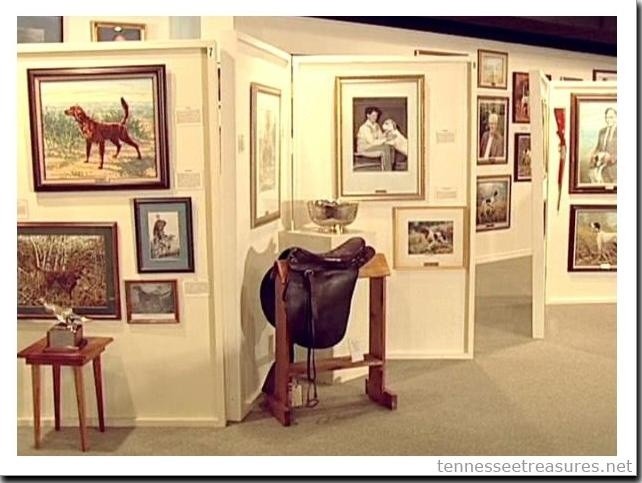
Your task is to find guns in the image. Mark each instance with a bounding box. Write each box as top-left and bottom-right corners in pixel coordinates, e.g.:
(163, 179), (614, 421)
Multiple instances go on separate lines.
(553, 107), (568, 212)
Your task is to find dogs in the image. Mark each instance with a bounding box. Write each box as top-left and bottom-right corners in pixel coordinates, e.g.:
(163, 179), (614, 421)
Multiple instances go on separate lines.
(31, 261), (88, 308)
(132, 286), (171, 312)
(372, 117), (408, 155)
(588, 221), (617, 261)
(413, 225), (448, 251)
(65, 96), (141, 170)
(477, 189), (499, 224)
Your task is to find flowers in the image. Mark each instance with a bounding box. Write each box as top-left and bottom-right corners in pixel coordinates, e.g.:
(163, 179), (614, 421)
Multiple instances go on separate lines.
(37, 260), (92, 323)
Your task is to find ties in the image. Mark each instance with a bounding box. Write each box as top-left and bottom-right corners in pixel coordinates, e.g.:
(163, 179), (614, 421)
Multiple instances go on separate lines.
(606, 127), (611, 152)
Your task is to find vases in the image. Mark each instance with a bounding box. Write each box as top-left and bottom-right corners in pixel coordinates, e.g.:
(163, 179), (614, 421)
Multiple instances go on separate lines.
(43, 323), (87, 354)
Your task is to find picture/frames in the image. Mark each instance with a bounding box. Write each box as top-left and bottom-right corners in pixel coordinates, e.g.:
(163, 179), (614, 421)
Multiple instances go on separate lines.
(90, 21), (149, 41)
(124, 280), (180, 325)
(17, 221), (122, 318)
(27, 65), (169, 191)
(250, 46), (618, 271)
(134, 198), (195, 273)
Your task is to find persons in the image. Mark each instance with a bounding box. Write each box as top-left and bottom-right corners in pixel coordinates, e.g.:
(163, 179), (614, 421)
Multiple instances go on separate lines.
(589, 107), (617, 184)
(356, 107), (395, 171)
(479, 113), (503, 157)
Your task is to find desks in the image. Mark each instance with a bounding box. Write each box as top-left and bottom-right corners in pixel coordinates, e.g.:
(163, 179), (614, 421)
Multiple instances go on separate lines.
(17, 338), (114, 452)
(264, 253), (398, 427)
